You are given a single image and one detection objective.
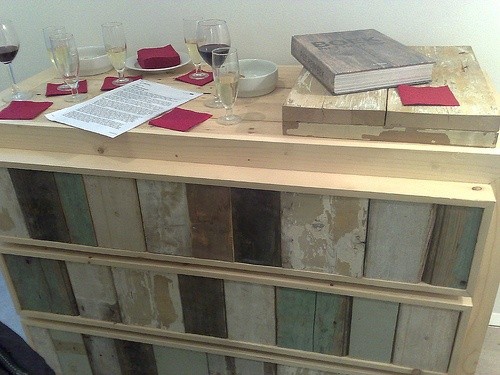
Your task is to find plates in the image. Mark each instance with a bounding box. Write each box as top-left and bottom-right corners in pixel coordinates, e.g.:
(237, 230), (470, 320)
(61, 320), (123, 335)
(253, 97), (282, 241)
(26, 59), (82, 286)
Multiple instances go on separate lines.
(125, 52), (192, 71)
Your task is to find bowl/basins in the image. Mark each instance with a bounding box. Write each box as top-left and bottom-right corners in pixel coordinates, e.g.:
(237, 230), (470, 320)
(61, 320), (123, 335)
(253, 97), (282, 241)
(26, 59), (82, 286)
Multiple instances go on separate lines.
(219, 59), (278, 98)
(78, 46), (113, 76)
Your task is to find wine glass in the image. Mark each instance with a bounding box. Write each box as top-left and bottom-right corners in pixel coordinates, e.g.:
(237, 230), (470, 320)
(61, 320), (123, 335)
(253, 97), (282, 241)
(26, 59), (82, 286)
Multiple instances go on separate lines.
(212, 48), (241, 125)
(196, 19), (231, 108)
(49, 33), (87, 102)
(101, 22), (133, 87)
(183, 16), (210, 80)
(0, 18), (35, 103)
(43, 26), (65, 83)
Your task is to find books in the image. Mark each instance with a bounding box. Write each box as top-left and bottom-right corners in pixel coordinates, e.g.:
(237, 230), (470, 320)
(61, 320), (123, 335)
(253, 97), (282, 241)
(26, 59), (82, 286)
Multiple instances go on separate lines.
(291, 30), (434, 96)
